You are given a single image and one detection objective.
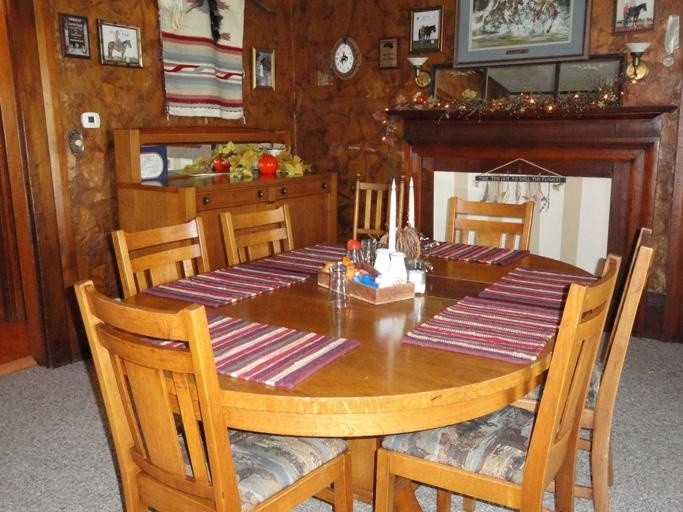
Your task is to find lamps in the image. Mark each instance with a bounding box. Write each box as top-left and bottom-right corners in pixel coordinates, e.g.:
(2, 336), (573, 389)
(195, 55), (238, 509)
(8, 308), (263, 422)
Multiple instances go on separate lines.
(406, 54), (431, 90)
(625, 41), (651, 80)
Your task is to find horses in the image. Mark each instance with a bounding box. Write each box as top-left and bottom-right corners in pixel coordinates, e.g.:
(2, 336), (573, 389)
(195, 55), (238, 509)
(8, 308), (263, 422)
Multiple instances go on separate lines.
(108, 40), (132, 58)
(629, 3), (647, 26)
(418, 25), (436, 40)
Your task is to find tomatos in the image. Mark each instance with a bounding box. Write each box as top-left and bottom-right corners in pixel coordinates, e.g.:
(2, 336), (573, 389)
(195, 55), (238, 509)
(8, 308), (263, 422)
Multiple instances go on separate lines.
(346, 239), (361, 250)
(214, 155), (231, 173)
(259, 154), (278, 175)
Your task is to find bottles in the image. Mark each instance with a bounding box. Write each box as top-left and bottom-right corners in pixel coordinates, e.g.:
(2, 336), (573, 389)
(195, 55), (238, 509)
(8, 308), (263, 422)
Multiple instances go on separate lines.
(389, 252), (405, 281)
(407, 259), (426, 294)
(374, 248), (388, 273)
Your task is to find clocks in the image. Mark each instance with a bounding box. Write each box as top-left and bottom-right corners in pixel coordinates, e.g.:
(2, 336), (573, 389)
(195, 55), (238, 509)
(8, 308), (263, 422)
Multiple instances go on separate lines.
(332, 37), (361, 80)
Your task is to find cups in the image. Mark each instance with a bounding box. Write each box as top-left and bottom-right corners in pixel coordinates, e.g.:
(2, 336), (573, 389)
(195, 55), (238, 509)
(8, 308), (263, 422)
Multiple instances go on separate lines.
(346, 248), (364, 264)
(362, 238), (376, 261)
(325, 264), (352, 308)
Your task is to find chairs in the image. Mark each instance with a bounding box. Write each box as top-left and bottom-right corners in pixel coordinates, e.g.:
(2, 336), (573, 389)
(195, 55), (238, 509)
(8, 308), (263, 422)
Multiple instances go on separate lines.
(111, 217), (210, 297)
(447, 195), (534, 251)
(373, 253), (623, 512)
(512, 225), (653, 512)
(352, 173), (407, 251)
(220, 203), (295, 265)
(74, 280), (353, 511)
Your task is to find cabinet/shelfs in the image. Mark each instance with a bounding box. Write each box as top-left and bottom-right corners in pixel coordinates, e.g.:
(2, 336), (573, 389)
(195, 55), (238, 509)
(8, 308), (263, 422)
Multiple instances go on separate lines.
(113, 126), (336, 270)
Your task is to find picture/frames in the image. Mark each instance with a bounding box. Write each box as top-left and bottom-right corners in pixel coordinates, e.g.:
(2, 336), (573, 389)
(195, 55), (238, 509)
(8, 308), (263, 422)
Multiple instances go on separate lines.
(98, 19), (142, 69)
(59, 12), (90, 61)
(453, 0), (592, 67)
(614, 0), (654, 33)
(410, 6), (444, 57)
(252, 45), (277, 89)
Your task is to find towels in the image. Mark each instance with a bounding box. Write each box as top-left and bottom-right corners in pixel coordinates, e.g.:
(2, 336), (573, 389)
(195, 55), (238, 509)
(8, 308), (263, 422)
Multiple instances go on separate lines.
(148, 265), (309, 308)
(420, 241), (530, 267)
(403, 294), (561, 367)
(483, 265), (598, 312)
(143, 313), (358, 387)
(251, 241), (351, 275)
